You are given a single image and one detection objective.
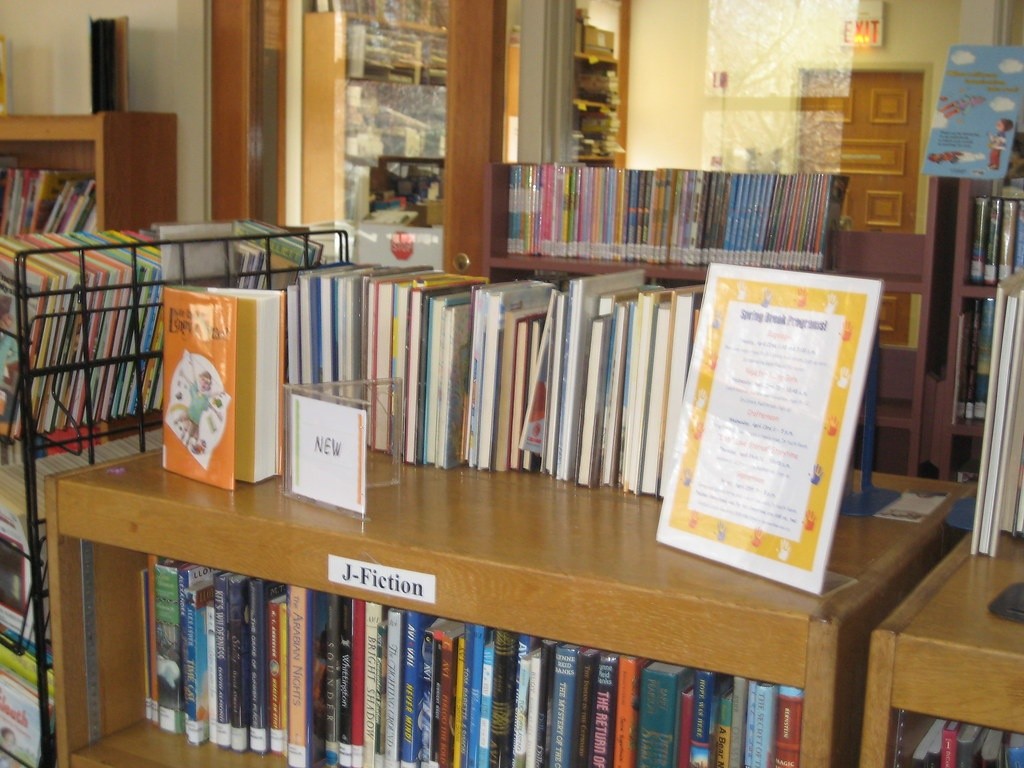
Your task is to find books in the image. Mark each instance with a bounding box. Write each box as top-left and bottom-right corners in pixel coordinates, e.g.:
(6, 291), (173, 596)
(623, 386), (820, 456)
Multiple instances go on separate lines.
(506, 164), (851, 274)
(0, 217), (323, 439)
(952, 184), (1024, 560)
(140, 553), (805, 768)
(899, 715), (1024, 768)
(162, 282), (285, 491)
(287, 264), (706, 503)
(570, 69), (626, 155)
(0, 509), (56, 768)
(0, 163), (98, 238)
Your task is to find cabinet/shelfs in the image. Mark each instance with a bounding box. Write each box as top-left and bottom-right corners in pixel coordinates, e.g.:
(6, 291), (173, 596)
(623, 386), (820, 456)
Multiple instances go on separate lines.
(0, 2), (1024, 767)
